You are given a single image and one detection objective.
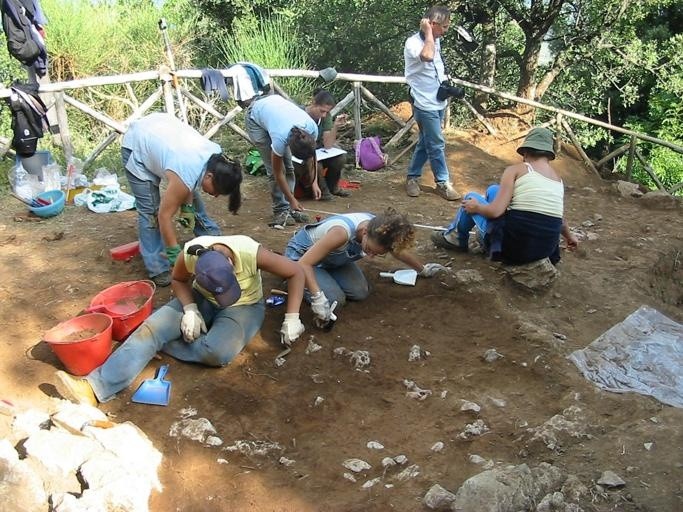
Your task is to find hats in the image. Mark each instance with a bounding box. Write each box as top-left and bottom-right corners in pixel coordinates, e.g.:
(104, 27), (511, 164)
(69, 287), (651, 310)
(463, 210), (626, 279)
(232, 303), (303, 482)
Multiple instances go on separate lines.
(195, 251), (241, 308)
(516, 127), (555, 159)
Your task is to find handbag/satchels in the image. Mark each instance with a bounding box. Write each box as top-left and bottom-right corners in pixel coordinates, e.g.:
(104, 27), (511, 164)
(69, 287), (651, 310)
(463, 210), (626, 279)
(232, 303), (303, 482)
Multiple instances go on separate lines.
(0, 0), (41, 66)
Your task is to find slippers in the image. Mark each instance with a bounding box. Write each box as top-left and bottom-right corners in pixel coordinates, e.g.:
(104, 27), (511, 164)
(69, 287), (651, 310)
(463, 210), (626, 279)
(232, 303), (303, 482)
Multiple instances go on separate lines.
(332, 188), (349, 196)
(430, 232), (461, 251)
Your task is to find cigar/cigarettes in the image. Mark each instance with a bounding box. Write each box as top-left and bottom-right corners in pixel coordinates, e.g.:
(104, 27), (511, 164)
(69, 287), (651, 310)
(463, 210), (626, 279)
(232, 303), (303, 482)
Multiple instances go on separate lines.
(318, 117), (322, 125)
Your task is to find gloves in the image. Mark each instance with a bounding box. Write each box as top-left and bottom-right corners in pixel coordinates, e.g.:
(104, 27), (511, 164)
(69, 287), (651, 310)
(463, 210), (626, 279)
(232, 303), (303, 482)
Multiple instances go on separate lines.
(176, 204), (195, 234)
(313, 301), (337, 329)
(165, 245), (182, 266)
(281, 313), (305, 345)
(181, 303), (208, 343)
(419, 263), (448, 278)
(310, 292), (331, 320)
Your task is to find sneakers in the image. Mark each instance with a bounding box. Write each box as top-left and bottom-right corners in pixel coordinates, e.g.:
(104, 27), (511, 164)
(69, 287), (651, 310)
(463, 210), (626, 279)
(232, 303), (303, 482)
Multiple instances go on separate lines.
(434, 179), (461, 200)
(289, 210), (308, 222)
(153, 273), (171, 287)
(273, 210), (296, 226)
(55, 371), (99, 408)
(405, 176), (420, 196)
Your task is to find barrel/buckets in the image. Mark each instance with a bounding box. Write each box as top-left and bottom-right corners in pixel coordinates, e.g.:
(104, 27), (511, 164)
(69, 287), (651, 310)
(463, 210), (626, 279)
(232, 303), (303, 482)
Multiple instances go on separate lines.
(90, 279), (156, 343)
(40, 312), (114, 376)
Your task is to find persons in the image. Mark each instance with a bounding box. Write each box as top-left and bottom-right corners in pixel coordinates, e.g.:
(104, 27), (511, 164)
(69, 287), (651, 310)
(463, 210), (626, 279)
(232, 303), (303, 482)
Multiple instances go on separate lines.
(121, 112), (243, 288)
(289, 90), (350, 199)
(53, 235), (306, 407)
(245, 93), (318, 226)
(429, 129), (580, 267)
(283, 208), (448, 326)
(406, 5), (463, 200)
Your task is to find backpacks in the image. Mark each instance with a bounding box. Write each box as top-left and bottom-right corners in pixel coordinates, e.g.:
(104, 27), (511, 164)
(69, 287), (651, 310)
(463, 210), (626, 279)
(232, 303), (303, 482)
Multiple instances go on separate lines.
(355, 137), (386, 172)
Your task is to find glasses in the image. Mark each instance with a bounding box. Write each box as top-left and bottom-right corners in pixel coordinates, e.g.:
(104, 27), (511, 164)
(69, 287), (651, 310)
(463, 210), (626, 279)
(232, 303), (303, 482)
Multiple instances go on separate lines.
(362, 246), (386, 260)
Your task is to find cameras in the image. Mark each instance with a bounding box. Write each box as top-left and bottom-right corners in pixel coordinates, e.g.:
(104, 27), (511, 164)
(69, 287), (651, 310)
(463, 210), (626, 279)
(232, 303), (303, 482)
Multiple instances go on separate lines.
(437, 81), (465, 101)
